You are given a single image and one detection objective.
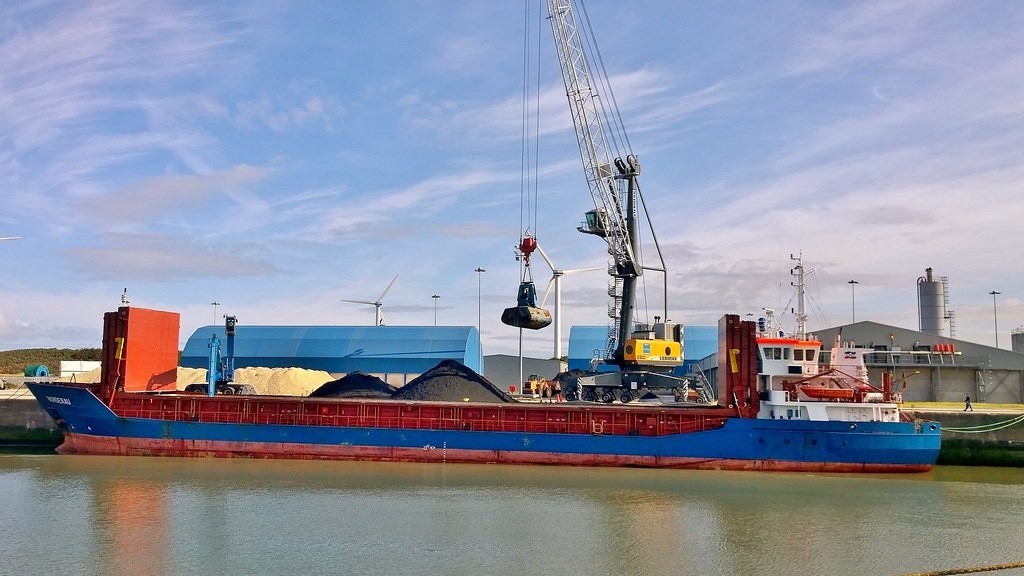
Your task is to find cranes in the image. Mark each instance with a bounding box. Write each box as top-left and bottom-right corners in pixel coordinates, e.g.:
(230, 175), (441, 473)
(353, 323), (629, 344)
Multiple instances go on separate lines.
(511, 0), (691, 405)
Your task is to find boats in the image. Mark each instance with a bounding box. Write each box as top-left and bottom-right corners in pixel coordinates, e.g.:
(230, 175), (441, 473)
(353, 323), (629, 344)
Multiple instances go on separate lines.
(23, 298), (947, 475)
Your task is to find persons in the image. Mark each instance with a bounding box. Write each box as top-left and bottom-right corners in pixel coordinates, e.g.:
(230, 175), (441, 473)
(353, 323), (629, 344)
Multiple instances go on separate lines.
(914, 341), (920, 351)
(890, 333), (895, 346)
(554, 378), (563, 404)
(964, 396), (973, 411)
